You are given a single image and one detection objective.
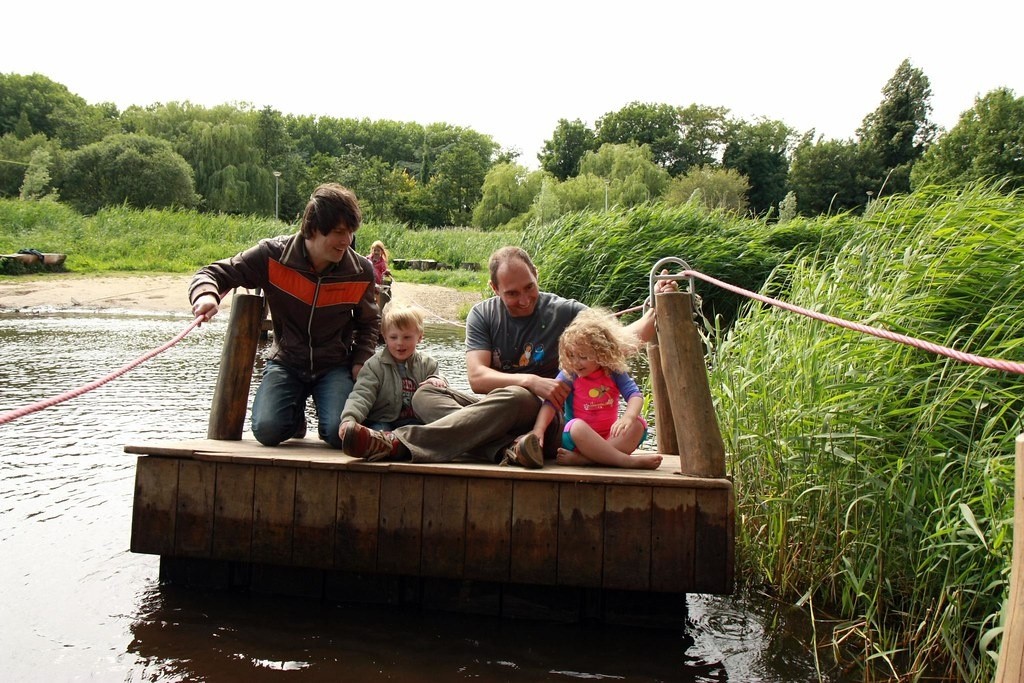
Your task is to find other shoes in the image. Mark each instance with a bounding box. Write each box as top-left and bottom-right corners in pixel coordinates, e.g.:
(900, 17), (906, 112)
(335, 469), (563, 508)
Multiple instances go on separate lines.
(292, 416), (306, 439)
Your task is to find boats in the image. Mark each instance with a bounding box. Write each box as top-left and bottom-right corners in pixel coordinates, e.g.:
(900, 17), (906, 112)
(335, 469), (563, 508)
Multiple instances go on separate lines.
(259, 269), (393, 344)
(123, 289), (736, 647)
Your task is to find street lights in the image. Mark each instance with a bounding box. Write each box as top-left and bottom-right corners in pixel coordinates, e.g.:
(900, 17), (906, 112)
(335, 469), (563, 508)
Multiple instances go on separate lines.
(273, 172), (282, 219)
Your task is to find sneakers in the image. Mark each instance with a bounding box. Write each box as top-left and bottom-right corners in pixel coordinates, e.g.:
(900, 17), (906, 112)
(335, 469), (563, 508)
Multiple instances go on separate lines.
(342, 421), (399, 462)
(498, 434), (543, 468)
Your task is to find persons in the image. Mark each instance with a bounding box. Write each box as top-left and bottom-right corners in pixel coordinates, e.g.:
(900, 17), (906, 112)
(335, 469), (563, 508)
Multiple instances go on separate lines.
(343, 246), (678, 468)
(188, 184), (382, 446)
(514, 309), (663, 469)
(339, 306), (449, 440)
(366, 240), (388, 299)
